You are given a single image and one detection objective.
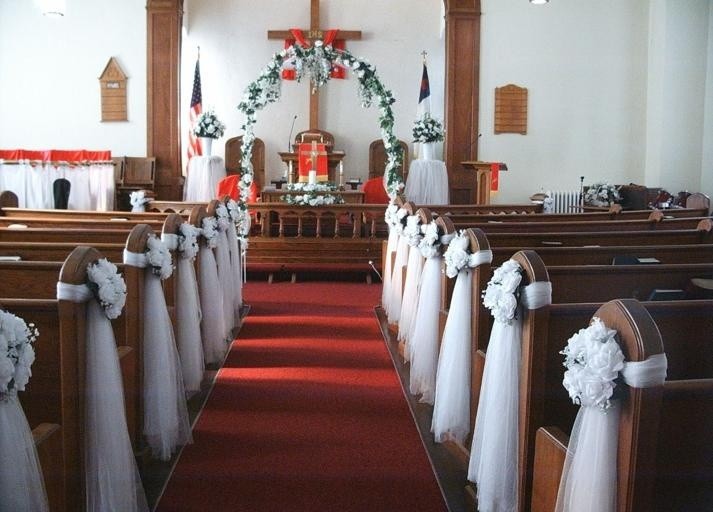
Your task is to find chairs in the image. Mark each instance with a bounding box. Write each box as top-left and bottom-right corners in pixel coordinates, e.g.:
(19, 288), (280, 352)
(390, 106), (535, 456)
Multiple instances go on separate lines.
(368, 138), (407, 187)
(293, 130), (333, 153)
(114, 157), (157, 212)
(225, 134), (265, 198)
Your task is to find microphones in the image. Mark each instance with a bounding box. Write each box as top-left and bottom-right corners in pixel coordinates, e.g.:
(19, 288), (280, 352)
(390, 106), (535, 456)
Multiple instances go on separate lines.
(289, 116), (297, 152)
(462, 134), (482, 161)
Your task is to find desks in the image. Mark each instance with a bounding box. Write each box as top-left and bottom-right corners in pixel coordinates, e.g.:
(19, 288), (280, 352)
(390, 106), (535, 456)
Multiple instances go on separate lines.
(458, 159), (508, 204)
(275, 150), (345, 186)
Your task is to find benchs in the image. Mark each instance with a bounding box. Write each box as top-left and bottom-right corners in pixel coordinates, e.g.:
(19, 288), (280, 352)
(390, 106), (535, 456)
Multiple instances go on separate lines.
(432, 227), (713, 459)
(0, 246), (133, 511)
(466, 250), (713, 512)
(530, 299), (713, 512)
(0, 309), (64, 512)
(1, 225), (195, 462)
